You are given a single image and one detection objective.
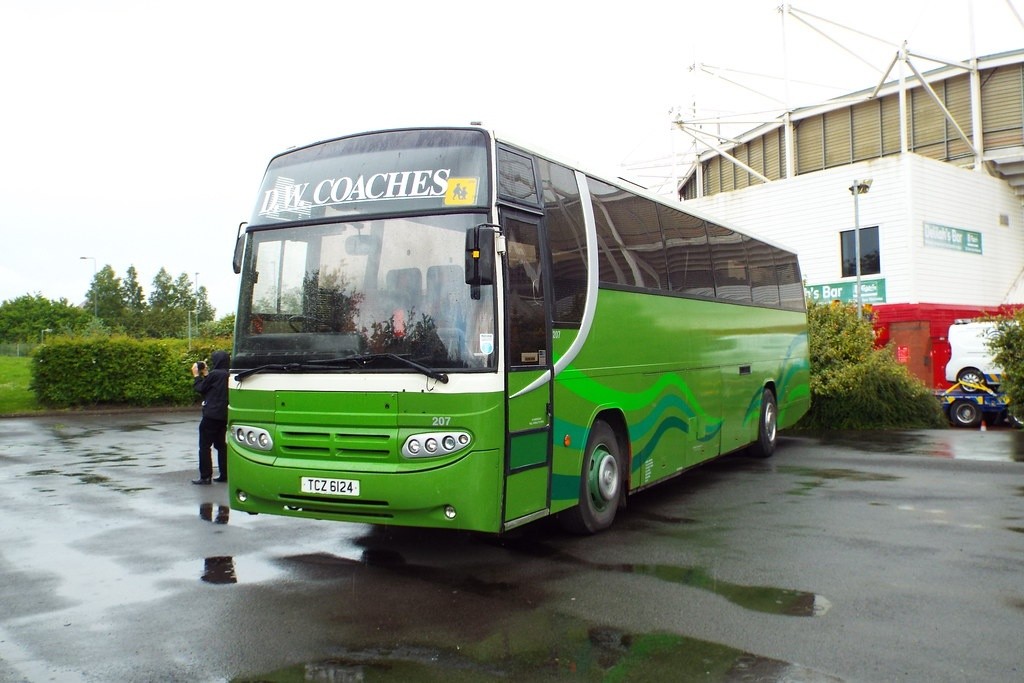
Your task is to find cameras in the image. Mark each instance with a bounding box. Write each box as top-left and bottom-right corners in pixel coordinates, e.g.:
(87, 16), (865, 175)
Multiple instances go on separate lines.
(197, 362), (205, 371)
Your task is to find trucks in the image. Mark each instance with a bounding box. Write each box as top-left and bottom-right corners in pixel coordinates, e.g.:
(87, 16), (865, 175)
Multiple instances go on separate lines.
(865, 303), (1024, 428)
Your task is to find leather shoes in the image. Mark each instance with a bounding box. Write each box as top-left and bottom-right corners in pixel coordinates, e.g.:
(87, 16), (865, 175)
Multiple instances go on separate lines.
(192, 476), (211, 485)
(213, 477), (227, 482)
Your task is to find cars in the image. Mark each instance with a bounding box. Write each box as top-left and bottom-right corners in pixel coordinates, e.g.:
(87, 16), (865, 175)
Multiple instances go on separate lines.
(944, 317), (1024, 391)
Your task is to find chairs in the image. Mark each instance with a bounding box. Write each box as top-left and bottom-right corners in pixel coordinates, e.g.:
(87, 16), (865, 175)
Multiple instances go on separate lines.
(385, 257), (589, 341)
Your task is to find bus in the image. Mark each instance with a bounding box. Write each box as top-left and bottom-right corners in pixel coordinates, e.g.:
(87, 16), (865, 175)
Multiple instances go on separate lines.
(223, 122), (815, 540)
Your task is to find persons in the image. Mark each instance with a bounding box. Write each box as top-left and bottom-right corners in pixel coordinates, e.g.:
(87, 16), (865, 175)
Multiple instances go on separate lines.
(191, 350), (230, 484)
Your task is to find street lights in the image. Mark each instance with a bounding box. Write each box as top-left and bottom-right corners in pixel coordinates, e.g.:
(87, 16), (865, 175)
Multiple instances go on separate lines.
(79, 256), (98, 317)
(194, 272), (199, 335)
(848, 178), (874, 316)
